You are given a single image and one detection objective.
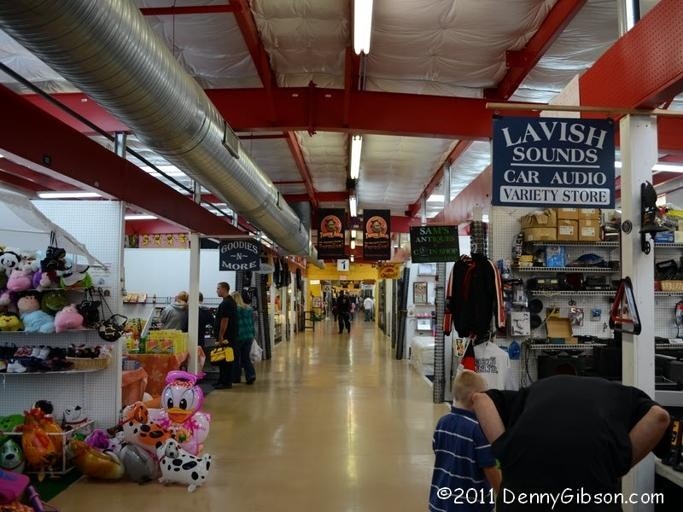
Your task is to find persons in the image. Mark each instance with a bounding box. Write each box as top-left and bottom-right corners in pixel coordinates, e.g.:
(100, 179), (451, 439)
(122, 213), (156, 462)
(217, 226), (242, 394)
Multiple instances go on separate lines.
(196, 291), (214, 349)
(331, 303), (339, 321)
(424, 367), (502, 511)
(325, 219), (337, 232)
(331, 294), (364, 313)
(471, 374), (671, 511)
(229, 290), (256, 385)
(158, 291), (188, 333)
(348, 299), (355, 321)
(336, 289), (351, 334)
(362, 295), (373, 322)
(209, 282), (238, 390)
(368, 219), (384, 233)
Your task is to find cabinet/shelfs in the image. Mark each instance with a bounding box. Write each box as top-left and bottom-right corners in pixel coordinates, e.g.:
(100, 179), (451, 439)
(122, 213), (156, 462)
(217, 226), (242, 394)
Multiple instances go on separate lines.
(0, 274), (103, 383)
(514, 239), (683, 351)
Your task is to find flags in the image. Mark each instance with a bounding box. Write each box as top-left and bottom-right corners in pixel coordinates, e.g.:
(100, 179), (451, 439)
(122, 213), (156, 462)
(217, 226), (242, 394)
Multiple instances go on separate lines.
(0, 244), (89, 339)
(0, 369), (214, 496)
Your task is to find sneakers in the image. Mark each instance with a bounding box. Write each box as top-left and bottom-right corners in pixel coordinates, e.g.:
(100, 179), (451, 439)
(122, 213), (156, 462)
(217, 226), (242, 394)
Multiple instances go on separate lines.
(246, 375), (255, 384)
(232, 378), (241, 383)
(0, 344), (101, 373)
(213, 381), (232, 389)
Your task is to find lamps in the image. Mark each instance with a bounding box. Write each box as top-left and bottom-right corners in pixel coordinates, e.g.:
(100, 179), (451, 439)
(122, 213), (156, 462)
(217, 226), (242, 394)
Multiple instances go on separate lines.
(349, 133), (361, 183)
(348, 197), (357, 263)
(352, 0), (372, 55)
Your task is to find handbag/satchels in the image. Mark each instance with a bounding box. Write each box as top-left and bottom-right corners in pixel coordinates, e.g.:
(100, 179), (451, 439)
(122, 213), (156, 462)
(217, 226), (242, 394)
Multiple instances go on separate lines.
(210, 347), (235, 362)
(76, 300), (101, 326)
(46, 246), (72, 270)
(98, 314), (128, 341)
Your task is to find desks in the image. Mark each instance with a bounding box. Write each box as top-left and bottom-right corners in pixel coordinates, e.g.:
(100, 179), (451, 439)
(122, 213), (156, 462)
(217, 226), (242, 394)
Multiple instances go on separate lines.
(303, 311), (314, 332)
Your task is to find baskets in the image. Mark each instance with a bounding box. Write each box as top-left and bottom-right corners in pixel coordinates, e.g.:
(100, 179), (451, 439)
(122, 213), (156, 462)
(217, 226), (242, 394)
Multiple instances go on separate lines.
(66, 356), (107, 369)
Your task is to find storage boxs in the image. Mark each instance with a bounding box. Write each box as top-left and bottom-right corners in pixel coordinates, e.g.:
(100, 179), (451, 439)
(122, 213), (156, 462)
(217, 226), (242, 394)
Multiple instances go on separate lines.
(520, 207), (601, 242)
